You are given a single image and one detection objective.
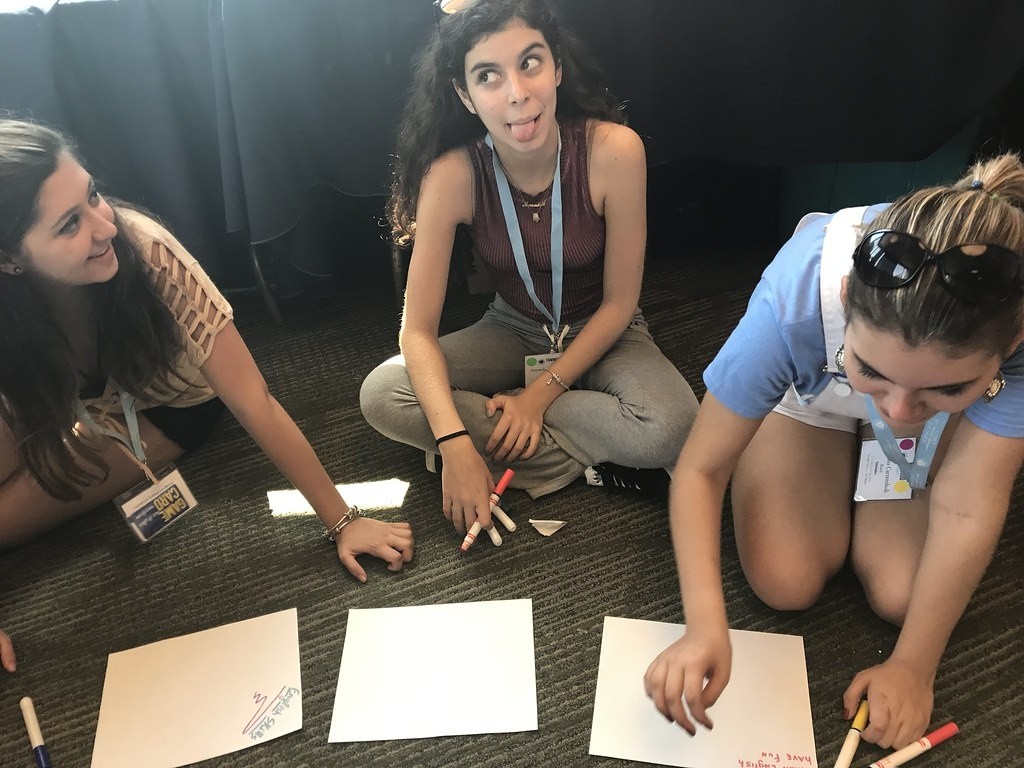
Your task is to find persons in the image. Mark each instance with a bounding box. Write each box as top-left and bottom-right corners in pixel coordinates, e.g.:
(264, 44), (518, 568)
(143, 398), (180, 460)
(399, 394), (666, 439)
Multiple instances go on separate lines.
(644, 148), (1024, 752)
(0, 110), (414, 675)
(357, 0), (700, 537)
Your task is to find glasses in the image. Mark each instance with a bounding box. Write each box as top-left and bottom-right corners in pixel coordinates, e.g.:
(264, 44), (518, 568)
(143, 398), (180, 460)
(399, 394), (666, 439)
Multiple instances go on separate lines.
(432, 0), (485, 42)
(852, 229), (1024, 313)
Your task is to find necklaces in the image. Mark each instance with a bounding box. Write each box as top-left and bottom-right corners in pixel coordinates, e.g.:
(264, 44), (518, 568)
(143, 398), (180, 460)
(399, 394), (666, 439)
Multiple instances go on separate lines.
(497, 158), (558, 222)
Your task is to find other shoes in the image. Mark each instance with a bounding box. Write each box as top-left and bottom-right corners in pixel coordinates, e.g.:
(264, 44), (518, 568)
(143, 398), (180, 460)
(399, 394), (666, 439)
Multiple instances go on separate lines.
(593, 461), (672, 498)
(425, 451), (443, 474)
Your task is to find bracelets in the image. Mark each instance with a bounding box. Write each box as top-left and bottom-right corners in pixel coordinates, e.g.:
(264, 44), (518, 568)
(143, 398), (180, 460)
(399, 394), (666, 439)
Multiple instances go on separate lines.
(321, 505), (364, 540)
(543, 366), (570, 390)
(435, 428), (470, 446)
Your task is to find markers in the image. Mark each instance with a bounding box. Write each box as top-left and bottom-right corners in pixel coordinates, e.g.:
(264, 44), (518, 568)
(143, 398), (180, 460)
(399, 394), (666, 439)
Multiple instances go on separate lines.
(460, 469), (516, 554)
(834, 698), (870, 768)
(867, 720), (960, 768)
(18, 696), (53, 768)
